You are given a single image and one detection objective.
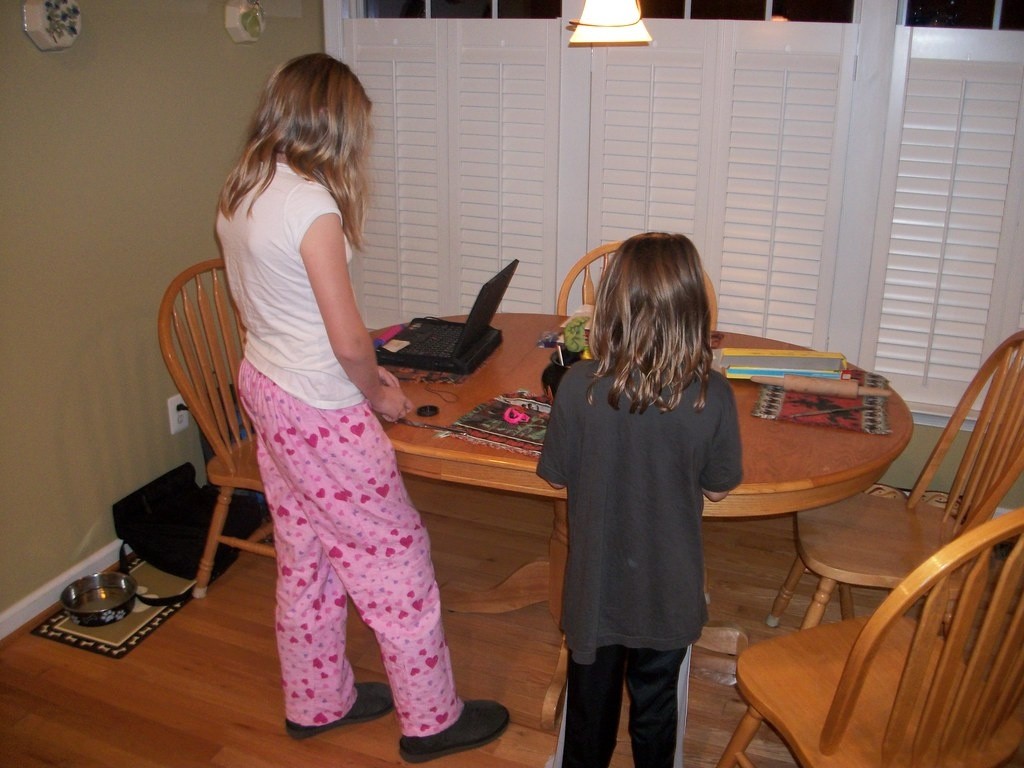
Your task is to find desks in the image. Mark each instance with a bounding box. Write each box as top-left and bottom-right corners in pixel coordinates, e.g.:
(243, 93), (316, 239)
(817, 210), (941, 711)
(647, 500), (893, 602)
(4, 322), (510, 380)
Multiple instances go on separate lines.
(368, 312), (914, 735)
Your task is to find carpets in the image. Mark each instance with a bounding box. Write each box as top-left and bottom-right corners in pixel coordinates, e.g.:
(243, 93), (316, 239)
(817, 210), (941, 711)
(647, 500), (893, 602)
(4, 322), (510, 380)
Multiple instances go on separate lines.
(29, 555), (197, 657)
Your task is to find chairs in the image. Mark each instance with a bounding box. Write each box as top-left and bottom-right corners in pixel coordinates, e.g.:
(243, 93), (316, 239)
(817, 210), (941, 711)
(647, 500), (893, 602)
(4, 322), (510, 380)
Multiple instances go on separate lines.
(157, 257), (282, 600)
(716, 505), (1024, 768)
(766, 328), (1024, 638)
(555, 238), (720, 330)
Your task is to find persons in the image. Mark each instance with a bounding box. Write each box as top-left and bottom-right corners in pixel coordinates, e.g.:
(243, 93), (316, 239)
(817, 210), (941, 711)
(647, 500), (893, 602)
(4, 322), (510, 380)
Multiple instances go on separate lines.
(215, 51), (510, 764)
(536, 232), (743, 768)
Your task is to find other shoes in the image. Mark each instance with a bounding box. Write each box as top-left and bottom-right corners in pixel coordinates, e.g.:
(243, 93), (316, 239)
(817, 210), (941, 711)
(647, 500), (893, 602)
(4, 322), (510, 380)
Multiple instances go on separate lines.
(398, 699), (509, 763)
(285, 681), (394, 740)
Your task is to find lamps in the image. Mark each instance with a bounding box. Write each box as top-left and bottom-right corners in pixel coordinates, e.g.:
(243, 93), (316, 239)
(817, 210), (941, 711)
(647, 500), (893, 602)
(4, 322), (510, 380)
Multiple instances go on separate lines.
(568, 0), (653, 43)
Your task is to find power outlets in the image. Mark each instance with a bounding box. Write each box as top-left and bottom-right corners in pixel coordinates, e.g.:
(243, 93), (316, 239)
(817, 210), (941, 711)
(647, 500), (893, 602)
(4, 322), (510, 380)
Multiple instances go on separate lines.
(168, 394), (190, 435)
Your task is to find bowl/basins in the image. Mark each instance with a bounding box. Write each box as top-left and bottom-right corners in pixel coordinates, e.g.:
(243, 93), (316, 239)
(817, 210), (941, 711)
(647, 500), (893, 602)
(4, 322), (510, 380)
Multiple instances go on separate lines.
(59, 571), (137, 627)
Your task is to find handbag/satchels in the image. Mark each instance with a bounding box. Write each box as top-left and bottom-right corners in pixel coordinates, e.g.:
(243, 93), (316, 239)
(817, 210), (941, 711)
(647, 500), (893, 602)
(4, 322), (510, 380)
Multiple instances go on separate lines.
(112, 462), (262, 607)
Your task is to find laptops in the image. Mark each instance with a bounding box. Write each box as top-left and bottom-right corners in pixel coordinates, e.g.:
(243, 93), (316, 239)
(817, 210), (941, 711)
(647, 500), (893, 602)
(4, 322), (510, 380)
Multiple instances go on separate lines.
(378, 259), (519, 364)
